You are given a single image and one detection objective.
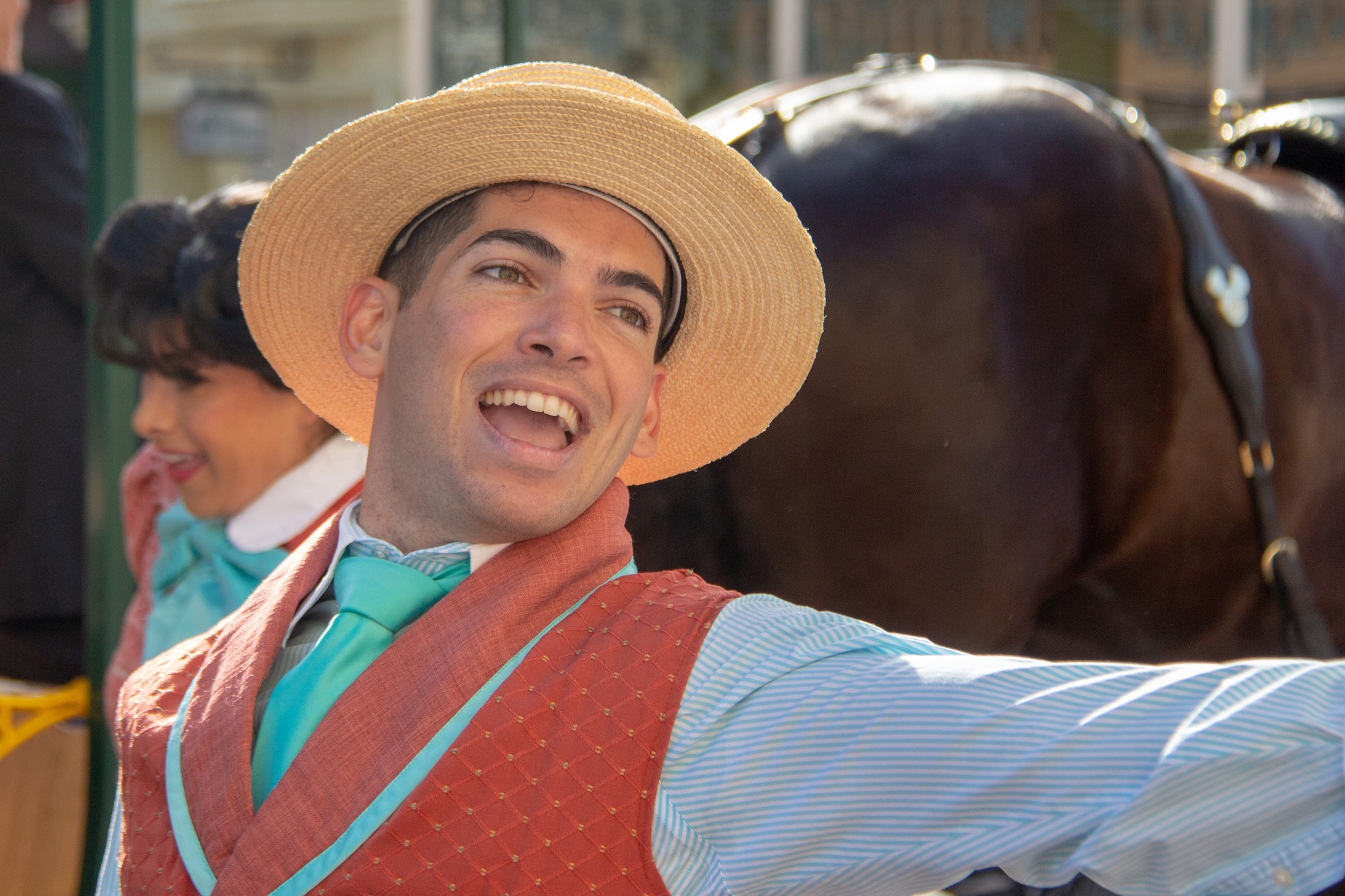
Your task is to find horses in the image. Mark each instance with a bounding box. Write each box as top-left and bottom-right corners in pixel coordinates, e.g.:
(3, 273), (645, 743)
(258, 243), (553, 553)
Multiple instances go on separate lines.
(625, 43), (1345, 663)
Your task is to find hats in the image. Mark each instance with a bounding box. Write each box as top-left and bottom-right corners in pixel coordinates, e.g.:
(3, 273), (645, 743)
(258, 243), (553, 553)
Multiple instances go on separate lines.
(237, 59), (831, 486)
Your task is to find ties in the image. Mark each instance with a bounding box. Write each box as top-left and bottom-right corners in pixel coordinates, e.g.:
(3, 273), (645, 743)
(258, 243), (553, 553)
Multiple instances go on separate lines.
(251, 555), (476, 816)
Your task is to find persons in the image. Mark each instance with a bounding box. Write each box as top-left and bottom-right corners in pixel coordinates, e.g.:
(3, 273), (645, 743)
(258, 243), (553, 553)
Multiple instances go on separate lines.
(85, 180), (368, 757)
(93, 59), (1345, 896)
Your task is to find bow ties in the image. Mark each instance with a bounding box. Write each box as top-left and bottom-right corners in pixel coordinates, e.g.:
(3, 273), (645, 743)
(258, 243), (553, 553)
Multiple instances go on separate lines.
(151, 506), (293, 613)
